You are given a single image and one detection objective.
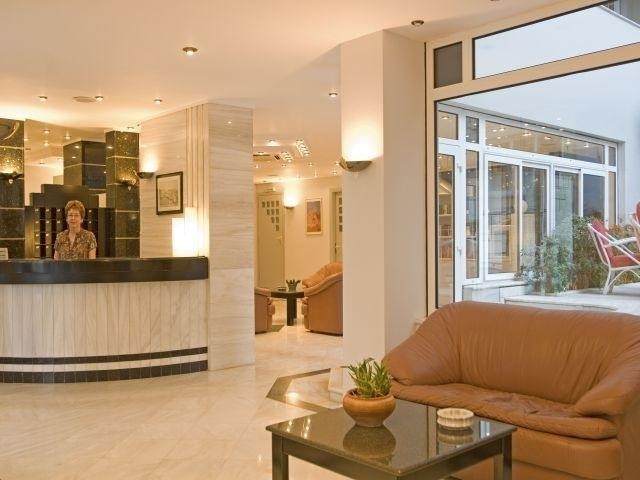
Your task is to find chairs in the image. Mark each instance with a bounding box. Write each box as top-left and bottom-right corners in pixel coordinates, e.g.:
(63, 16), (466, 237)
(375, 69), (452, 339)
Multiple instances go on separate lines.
(587, 217), (639, 296)
(253, 262), (343, 337)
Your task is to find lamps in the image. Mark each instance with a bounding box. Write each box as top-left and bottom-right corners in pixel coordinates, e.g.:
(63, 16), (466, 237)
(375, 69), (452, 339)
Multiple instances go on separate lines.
(133, 169), (153, 179)
(340, 157), (372, 172)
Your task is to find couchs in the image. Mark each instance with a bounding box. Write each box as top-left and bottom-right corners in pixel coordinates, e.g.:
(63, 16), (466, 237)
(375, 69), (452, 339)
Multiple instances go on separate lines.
(382, 300), (639, 480)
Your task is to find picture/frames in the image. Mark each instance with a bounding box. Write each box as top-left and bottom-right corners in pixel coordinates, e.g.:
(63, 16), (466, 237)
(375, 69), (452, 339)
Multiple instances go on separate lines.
(305, 199), (322, 235)
(156, 171), (183, 215)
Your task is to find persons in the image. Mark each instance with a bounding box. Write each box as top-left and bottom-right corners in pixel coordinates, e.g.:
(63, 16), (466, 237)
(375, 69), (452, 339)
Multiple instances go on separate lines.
(54, 201), (98, 260)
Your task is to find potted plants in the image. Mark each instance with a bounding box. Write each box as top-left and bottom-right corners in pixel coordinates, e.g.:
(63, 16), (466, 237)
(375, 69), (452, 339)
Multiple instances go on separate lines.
(340, 356), (396, 428)
(342, 423), (398, 466)
